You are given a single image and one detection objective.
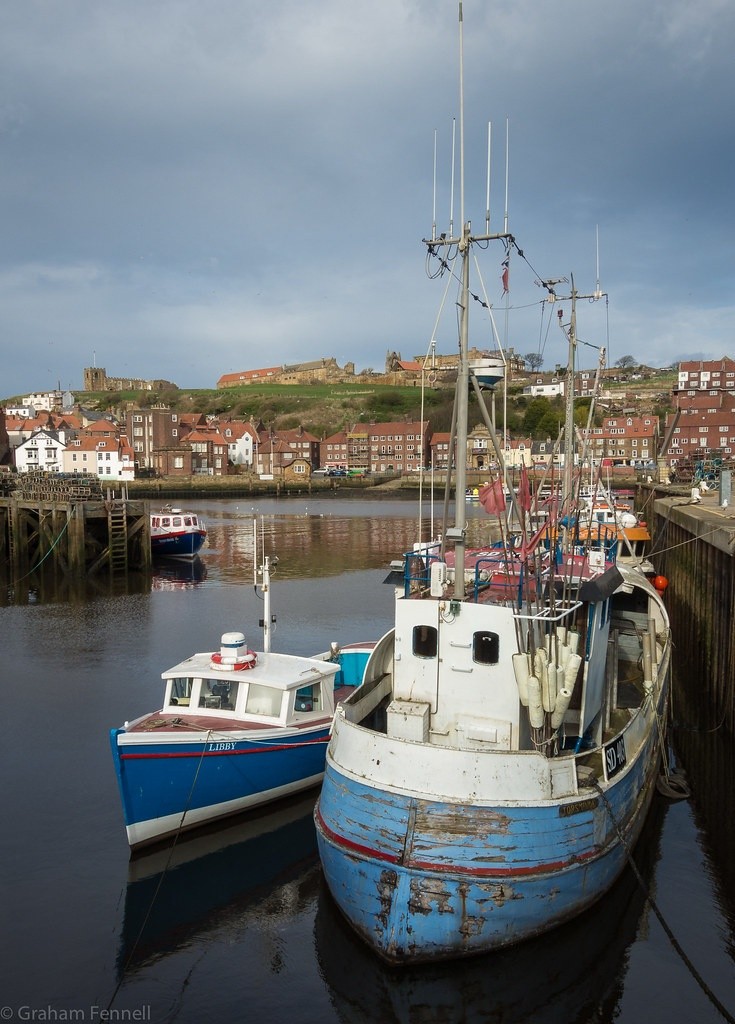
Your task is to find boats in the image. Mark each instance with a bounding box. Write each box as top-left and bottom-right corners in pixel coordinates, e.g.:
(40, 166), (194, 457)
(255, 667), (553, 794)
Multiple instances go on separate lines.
(152, 553), (208, 585)
(151, 508), (207, 553)
(312, 752), (677, 1024)
(115, 780), (322, 984)
(111, 632), (379, 853)
(312, 2), (674, 975)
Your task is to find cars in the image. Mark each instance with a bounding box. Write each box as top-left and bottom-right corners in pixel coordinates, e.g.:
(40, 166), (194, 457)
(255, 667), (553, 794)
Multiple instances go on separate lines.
(412, 466), (564, 471)
(312, 466), (367, 478)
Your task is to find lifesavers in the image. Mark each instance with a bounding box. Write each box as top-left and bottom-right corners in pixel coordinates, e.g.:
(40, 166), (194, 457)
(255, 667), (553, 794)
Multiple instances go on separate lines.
(105, 501), (115, 511)
(209, 649), (257, 671)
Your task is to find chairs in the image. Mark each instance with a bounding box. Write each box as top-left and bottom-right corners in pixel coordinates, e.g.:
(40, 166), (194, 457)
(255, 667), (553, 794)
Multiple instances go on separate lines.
(204, 695), (221, 708)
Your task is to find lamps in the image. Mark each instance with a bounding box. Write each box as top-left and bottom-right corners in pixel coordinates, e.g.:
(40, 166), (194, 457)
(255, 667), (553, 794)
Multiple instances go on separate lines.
(390, 561), (406, 572)
(445, 521), (468, 542)
(450, 601), (460, 614)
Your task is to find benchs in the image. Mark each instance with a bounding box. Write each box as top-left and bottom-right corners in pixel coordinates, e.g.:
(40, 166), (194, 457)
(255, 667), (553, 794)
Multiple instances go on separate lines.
(609, 610), (648, 662)
